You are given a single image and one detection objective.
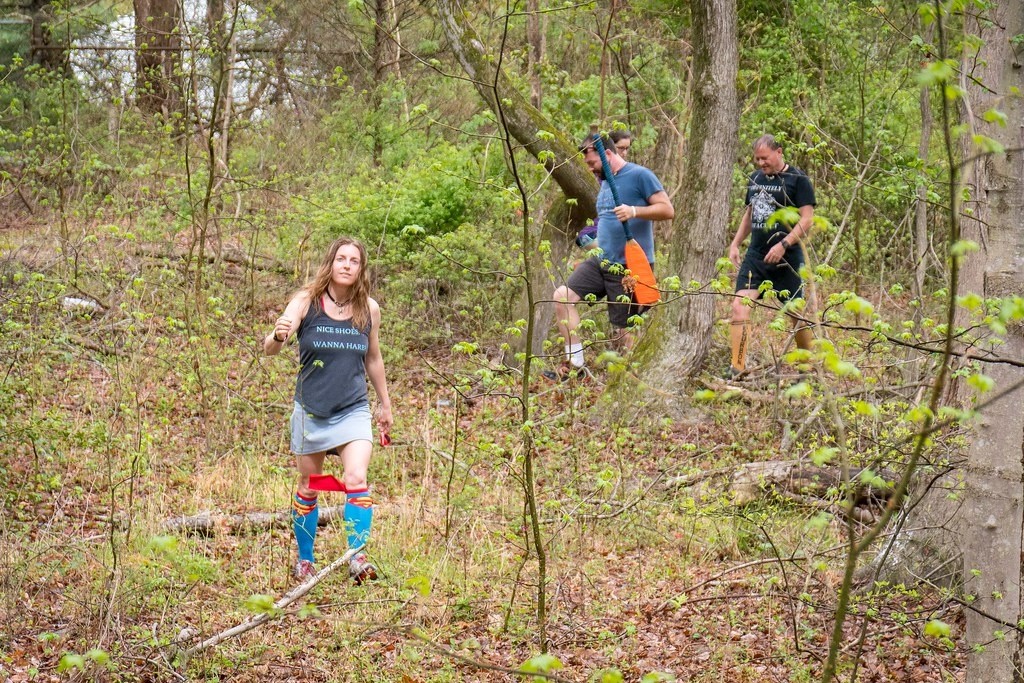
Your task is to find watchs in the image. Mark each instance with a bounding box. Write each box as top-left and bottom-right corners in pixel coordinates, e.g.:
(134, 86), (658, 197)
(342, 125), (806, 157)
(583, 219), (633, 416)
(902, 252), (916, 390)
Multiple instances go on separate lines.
(781, 240), (790, 249)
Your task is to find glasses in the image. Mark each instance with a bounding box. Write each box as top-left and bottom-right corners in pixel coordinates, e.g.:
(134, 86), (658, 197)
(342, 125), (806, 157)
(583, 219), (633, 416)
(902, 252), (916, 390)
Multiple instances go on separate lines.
(617, 146), (630, 152)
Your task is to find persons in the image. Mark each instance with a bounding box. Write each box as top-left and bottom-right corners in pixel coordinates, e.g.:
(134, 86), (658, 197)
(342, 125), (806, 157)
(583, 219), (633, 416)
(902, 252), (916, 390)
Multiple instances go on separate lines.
(719, 134), (818, 392)
(541, 131), (674, 384)
(264, 238), (394, 587)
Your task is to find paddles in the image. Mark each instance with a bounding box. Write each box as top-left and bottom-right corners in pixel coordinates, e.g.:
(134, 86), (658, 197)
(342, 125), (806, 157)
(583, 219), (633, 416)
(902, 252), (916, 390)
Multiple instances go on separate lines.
(591, 132), (660, 304)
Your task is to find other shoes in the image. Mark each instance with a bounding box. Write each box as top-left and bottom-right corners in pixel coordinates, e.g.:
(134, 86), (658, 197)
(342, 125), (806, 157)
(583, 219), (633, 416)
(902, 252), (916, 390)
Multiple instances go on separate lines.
(541, 361), (590, 385)
(708, 365), (749, 383)
(797, 368), (819, 384)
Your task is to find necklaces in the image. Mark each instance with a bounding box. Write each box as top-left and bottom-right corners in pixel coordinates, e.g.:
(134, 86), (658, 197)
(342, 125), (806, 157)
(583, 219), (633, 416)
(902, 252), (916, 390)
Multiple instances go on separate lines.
(325, 288), (351, 315)
(781, 163), (786, 171)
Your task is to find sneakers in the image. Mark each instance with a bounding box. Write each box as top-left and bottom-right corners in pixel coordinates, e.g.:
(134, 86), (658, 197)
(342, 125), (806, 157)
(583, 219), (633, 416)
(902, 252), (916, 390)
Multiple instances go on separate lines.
(295, 560), (312, 582)
(350, 554), (378, 588)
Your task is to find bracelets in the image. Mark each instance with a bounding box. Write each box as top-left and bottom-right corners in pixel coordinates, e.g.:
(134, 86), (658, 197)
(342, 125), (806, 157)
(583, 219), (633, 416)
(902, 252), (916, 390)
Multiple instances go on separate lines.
(273, 331), (288, 342)
(632, 206), (635, 218)
